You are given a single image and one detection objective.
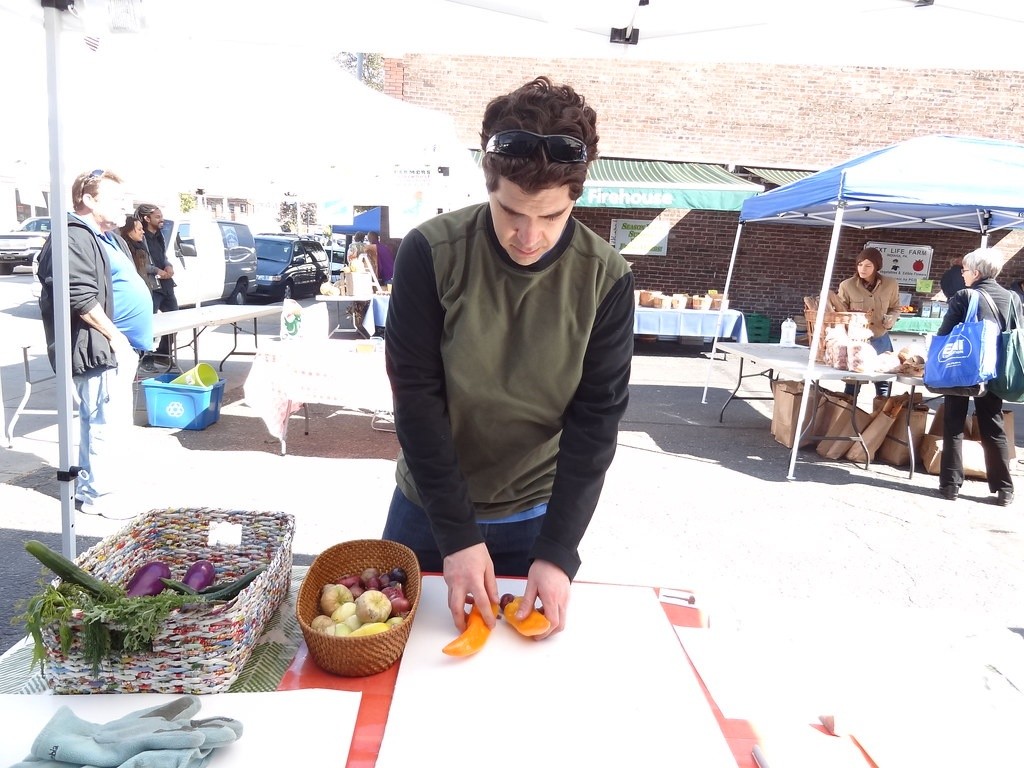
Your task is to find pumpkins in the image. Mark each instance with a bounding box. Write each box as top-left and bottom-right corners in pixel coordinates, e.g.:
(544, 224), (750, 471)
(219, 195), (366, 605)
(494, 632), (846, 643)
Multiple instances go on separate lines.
(320, 281), (341, 296)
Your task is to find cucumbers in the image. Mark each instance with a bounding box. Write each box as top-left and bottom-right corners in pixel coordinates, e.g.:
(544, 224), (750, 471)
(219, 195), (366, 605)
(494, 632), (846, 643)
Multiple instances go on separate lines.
(25, 540), (104, 597)
(196, 566), (265, 600)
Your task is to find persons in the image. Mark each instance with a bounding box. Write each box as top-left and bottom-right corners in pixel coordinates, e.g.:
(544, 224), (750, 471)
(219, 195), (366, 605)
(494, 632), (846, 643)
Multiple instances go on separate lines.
(120, 217), (161, 292)
(837, 247), (900, 396)
(382, 75), (636, 641)
(37, 170), (156, 519)
(367, 232), (379, 278)
(937, 247), (1022, 506)
(940, 254), (966, 300)
(347, 232), (371, 265)
(134, 203), (178, 372)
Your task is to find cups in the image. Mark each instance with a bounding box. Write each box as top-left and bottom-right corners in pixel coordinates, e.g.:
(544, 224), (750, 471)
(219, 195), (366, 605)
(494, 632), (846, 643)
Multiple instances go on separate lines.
(387, 284), (392, 292)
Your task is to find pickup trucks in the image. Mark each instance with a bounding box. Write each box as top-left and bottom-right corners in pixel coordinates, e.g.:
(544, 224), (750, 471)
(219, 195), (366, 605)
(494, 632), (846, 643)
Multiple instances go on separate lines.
(0, 216), (52, 275)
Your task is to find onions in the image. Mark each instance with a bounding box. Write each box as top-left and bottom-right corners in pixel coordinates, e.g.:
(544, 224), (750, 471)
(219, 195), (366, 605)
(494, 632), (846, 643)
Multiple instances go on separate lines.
(336, 575), (411, 618)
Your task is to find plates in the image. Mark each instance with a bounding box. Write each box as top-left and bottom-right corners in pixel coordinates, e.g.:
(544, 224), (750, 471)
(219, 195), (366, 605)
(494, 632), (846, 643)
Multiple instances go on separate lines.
(900, 312), (917, 318)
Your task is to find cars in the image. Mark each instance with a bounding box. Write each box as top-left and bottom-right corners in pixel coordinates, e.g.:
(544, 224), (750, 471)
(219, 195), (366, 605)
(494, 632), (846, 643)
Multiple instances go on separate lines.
(279, 232), (347, 246)
(322, 245), (346, 282)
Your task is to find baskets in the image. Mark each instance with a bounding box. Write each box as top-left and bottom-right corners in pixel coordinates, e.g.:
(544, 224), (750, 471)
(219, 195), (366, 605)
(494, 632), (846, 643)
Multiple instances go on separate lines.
(297, 539), (420, 677)
(804, 310), (867, 362)
(42, 506), (295, 695)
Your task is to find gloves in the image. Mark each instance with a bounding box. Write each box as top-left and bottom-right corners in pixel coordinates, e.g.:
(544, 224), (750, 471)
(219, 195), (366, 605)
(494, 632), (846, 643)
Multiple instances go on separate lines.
(12, 695), (244, 768)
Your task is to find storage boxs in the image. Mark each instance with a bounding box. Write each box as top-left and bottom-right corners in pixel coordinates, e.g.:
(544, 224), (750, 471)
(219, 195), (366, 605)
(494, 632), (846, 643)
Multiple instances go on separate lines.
(141, 373), (227, 430)
(744, 314), (770, 342)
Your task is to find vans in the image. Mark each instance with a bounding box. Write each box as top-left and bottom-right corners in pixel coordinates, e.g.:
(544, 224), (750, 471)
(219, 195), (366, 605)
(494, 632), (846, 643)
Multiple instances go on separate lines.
(215, 219), (258, 306)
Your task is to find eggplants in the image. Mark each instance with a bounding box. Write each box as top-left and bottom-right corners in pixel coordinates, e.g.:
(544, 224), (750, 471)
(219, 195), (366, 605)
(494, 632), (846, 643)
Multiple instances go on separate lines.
(180, 560), (214, 590)
(123, 561), (172, 598)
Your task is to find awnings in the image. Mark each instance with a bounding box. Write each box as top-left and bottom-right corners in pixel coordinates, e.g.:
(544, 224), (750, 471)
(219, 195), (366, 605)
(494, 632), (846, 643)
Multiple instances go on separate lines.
(575, 159), (765, 210)
(742, 166), (820, 186)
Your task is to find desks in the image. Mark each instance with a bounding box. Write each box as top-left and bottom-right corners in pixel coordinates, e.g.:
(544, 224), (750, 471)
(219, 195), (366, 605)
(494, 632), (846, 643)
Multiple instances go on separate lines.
(0, 564), (767, 768)
(362, 294), (389, 340)
(715, 342), (1024, 479)
(7, 305), (283, 447)
(634, 301), (749, 343)
(888, 318), (945, 333)
(315, 294), (372, 339)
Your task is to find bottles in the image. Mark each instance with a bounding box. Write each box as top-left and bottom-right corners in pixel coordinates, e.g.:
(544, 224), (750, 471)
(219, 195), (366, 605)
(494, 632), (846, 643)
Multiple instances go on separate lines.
(780, 314), (796, 346)
(350, 257), (357, 272)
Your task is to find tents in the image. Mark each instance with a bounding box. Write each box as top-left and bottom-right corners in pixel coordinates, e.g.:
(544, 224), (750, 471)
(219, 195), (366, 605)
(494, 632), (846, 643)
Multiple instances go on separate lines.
(0, 0), (1023, 564)
(330, 206), (381, 283)
(701, 131), (1024, 479)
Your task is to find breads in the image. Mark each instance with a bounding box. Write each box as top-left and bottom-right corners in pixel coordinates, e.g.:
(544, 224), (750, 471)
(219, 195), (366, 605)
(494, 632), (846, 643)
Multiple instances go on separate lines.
(897, 351), (924, 372)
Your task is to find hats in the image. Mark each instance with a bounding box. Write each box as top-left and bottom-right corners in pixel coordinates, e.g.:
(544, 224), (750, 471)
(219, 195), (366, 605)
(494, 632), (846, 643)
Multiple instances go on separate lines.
(856, 247), (882, 271)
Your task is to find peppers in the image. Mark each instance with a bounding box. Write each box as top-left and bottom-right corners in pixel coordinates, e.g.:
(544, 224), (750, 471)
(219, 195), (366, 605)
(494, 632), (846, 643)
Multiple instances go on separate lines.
(504, 597), (551, 636)
(442, 599), (500, 656)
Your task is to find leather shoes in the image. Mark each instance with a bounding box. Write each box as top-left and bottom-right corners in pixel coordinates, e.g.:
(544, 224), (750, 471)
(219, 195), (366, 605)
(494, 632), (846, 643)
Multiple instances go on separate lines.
(997, 490), (1014, 506)
(939, 483), (959, 500)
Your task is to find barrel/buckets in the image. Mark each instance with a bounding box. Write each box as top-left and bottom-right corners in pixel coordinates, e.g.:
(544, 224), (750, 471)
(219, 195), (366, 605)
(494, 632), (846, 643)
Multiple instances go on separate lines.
(169, 363), (219, 388)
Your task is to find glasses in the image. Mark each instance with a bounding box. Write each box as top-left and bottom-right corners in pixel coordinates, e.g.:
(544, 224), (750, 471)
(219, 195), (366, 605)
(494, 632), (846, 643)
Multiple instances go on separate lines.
(961, 269), (970, 274)
(485, 130), (588, 164)
(80, 170), (103, 202)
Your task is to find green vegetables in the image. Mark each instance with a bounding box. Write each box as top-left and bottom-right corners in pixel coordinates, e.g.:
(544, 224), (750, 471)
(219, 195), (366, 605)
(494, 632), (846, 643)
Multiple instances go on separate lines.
(9, 576), (208, 682)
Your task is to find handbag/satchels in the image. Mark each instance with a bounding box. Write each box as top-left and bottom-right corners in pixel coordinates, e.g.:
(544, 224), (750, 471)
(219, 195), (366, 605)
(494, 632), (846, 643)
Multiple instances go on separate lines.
(923, 287), (1024, 402)
(771, 377), (1016, 479)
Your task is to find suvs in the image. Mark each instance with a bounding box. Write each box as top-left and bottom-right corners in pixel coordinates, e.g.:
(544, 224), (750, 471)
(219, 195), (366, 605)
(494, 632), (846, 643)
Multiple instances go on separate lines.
(253, 233), (330, 303)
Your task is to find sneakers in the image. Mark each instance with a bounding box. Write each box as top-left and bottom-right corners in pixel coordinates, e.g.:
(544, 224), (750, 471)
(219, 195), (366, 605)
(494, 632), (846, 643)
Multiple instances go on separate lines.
(141, 358), (159, 373)
(155, 358), (176, 368)
(80, 491), (138, 519)
